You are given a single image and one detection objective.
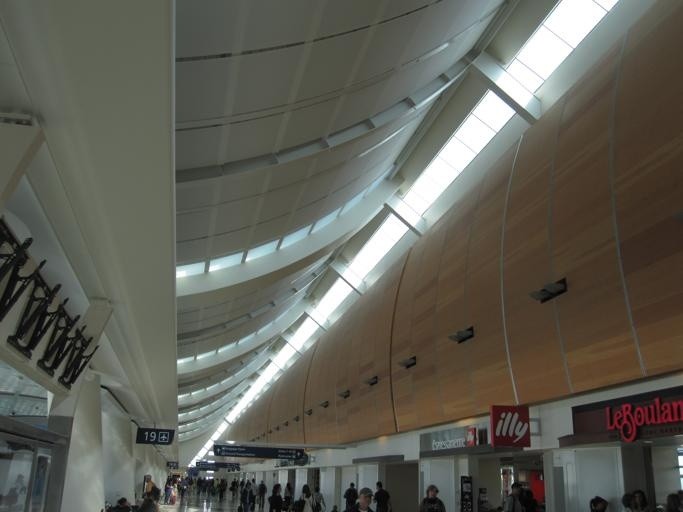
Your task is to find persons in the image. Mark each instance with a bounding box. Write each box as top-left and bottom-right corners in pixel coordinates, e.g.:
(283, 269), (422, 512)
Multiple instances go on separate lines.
(588, 488), (682, 511)
(162, 474), (338, 511)
(109, 496), (132, 511)
(137, 495), (158, 512)
(0, 464), (45, 512)
(343, 487), (374, 512)
(372, 481), (392, 512)
(487, 481), (545, 511)
(144, 473), (154, 502)
(418, 484), (445, 511)
(343, 481), (358, 510)
